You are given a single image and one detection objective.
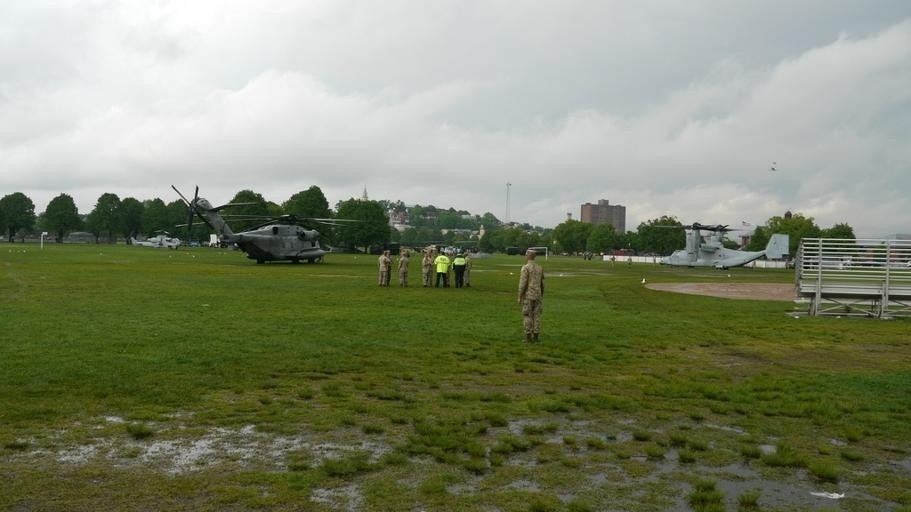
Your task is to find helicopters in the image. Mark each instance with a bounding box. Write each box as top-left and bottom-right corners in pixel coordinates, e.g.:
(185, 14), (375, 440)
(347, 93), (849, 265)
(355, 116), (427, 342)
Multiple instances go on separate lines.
(651, 222), (794, 271)
(170, 182), (368, 266)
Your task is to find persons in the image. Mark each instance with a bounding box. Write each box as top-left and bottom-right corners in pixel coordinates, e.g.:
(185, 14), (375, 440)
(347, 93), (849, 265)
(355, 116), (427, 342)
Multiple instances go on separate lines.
(375, 244), (473, 289)
(518, 250), (546, 342)
(550, 247), (634, 270)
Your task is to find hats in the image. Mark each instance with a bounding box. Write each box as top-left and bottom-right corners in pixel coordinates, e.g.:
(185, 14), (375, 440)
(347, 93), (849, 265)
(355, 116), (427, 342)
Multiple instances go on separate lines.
(525, 249), (537, 256)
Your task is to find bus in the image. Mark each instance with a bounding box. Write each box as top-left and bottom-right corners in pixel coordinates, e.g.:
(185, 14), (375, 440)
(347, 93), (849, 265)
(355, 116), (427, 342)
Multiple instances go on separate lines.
(811, 253), (859, 273)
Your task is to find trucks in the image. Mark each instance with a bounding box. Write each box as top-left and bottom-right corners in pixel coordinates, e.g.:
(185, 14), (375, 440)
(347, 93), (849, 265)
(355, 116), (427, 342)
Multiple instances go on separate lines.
(399, 245), (467, 255)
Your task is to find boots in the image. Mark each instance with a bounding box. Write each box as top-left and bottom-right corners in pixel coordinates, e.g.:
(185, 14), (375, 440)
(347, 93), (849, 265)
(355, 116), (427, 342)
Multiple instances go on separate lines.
(522, 333), (538, 343)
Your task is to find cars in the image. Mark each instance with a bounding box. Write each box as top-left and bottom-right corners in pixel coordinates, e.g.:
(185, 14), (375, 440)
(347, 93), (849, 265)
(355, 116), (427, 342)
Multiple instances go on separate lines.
(130, 236), (197, 249)
